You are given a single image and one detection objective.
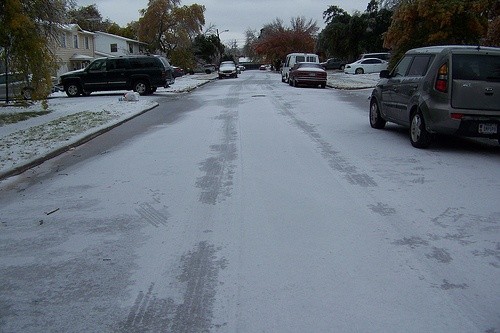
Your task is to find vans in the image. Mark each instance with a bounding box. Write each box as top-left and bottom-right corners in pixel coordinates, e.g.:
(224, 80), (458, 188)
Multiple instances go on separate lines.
(358, 53), (390, 63)
(282, 53), (319, 83)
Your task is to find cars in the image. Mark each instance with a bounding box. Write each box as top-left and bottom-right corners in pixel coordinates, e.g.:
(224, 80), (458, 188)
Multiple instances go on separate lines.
(0, 72), (51, 100)
(344, 58), (388, 75)
(288, 62), (327, 89)
(320, 58), (347, 71)
(218, 61), (245, 78)
(259, 65), (268, 70)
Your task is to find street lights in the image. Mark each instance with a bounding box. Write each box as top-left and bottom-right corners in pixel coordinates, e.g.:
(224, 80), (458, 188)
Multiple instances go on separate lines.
(217, 29), (229, 71)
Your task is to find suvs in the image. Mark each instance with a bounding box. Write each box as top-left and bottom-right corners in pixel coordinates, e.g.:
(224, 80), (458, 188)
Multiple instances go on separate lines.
(370, 46), (500, 148)
(187, 63), (215, 75)
(59, 55), (183, 98)
